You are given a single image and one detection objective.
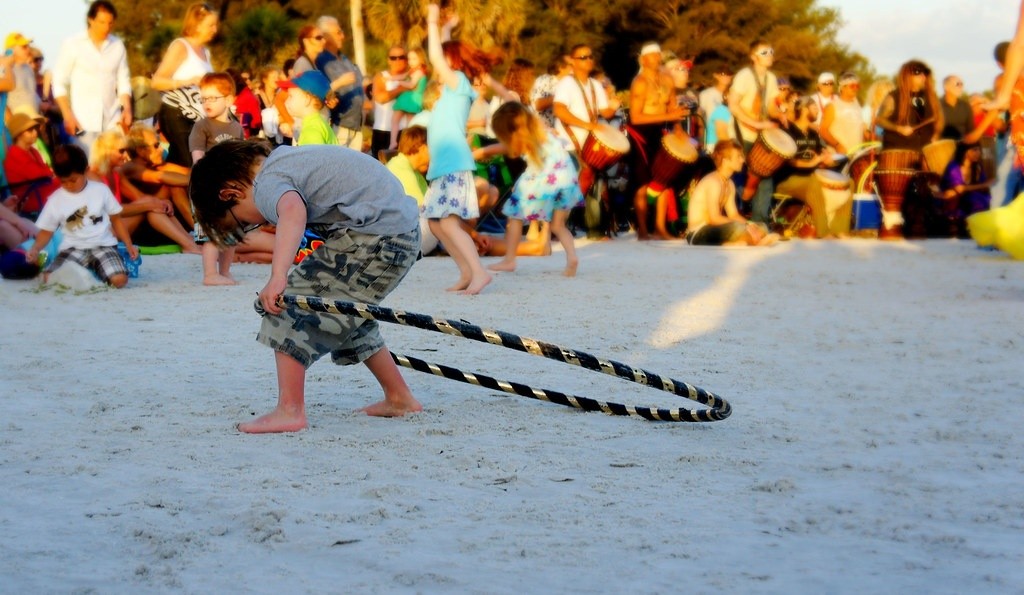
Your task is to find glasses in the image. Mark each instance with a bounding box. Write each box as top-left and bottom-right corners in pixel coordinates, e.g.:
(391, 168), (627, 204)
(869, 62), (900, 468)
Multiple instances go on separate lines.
(850, 84), (860, 89)
(574, 55), (594, 61)
(118, 148), (129, 154)
(822, 82), (834, 86)
(389, 55), (406, 60)
(33, 57), (44, 63)
(200, 95), (227, 103)
(911, 68), (929, 77)
(780, 87), (791, 91)
(243, 77), (252, 81)
(305, 35), (324, 40)
(755, 49), (774, 56)
(218, 187), (261, 233)
(144, 142), (159, 148)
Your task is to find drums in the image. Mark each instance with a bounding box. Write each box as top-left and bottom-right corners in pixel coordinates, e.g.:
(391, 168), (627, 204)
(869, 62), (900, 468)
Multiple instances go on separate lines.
(814, 167), (851, 226)
(741, 125), (799, 204)
(578, 123), (631, 197)
(878, 148), (920, 240)
(921, 138), (967, 200)
(645, 134), (699, 205)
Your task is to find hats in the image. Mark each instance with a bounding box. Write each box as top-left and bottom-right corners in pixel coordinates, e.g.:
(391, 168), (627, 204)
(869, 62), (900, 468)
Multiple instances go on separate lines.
(818, 72), (834, 84)
(4, 32), (32, 50)
(5, 113), (39, 142)
(837, 73), (860, 86)
(275, 70), (330, 101)
(715, 65), (734, 75)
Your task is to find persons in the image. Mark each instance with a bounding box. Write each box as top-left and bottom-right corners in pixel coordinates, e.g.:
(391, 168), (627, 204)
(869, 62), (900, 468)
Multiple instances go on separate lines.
(473, 101), (584, 277)
(275, 70), (338, 264)
(0, 0), (1024, 265)
(686, 139), (777, 247)
(190, 138), (422, 433)
(421, 0), (505, 293)
(27, 145), (138, 288)
(189, 71), (243, 286)
